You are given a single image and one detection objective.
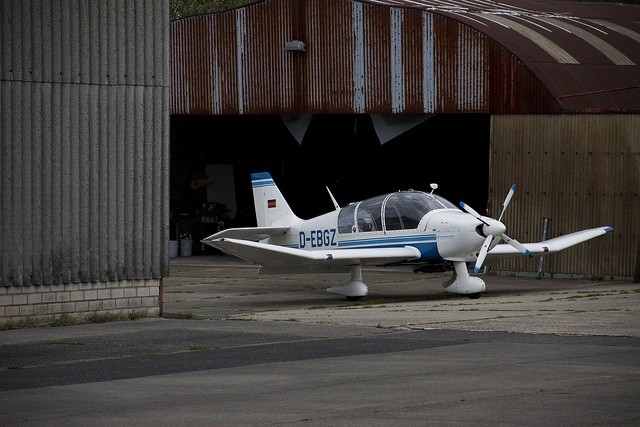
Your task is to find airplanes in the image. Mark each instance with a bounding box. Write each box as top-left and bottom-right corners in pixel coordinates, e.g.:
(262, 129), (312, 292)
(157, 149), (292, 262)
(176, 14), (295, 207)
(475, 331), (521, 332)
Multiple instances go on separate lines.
(200, 172), (612, 300)
